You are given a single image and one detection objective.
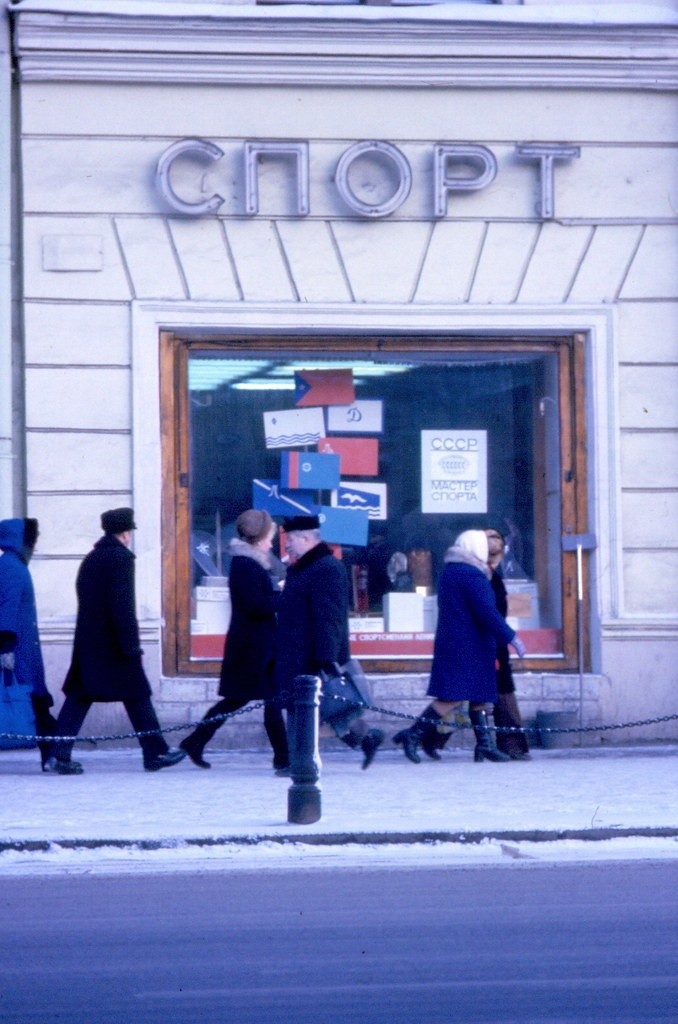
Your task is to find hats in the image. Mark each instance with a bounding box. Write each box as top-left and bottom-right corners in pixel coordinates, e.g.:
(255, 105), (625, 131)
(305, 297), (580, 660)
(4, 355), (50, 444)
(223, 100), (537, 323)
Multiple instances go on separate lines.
(101, 507), (138, 535)
(236, 508), (272, 544)
(281, 514), (321, 535)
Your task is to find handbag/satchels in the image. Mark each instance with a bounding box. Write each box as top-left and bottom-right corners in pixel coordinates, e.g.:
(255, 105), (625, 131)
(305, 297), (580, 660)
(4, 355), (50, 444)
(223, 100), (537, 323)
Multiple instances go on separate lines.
(0, 666), (38, 752)
(322, 657), (376, 725)
(318, 661), (364, 726)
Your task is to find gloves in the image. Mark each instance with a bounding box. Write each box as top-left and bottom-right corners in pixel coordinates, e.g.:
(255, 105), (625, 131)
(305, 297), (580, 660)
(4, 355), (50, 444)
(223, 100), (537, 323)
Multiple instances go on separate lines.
(511, 636), (526, 660)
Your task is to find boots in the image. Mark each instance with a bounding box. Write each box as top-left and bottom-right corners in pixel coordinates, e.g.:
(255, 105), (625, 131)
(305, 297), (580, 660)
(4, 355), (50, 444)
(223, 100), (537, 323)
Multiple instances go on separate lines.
(468, 709), (511, 762)
(392, 703), (442, 764)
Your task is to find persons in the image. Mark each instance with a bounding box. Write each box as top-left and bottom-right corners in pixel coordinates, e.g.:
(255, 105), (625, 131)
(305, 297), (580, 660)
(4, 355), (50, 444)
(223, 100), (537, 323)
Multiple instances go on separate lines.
(390, 528), (513, 765)
(0, 516), (85, 778)
(417, 527), (534, 762)
(42, 507), (188, 776)
(266, 513), (387, 776)
(176, 508), (292, 770)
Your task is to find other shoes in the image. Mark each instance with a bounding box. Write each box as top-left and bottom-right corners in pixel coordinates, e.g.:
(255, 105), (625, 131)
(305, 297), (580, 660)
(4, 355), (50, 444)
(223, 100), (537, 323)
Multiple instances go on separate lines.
(41, 761), (81, 772)
(511, 753), (531, 760)
(422, 746), (441, 761)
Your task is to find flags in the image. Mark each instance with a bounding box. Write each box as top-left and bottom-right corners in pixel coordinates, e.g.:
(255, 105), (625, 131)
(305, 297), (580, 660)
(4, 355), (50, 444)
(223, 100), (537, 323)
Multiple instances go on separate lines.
(279, 449), (342, 491)
(293, 368), (355, 407)
(317, 505), (371, 548)
(259, 406), (326, 450)
(251, 478), (320, 518)
(331, 479), (390, 521)
(327, 397), (384, 435)
(319, 437), (380, 478)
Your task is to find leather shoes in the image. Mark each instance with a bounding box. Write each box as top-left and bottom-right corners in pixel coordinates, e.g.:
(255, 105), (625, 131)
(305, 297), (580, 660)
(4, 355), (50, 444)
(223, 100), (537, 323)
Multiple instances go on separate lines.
(361, 728), (383, 769)
(44, 756), (83, 775)
(180, 739), (211, 768)
(144, 750), (186, 770)
